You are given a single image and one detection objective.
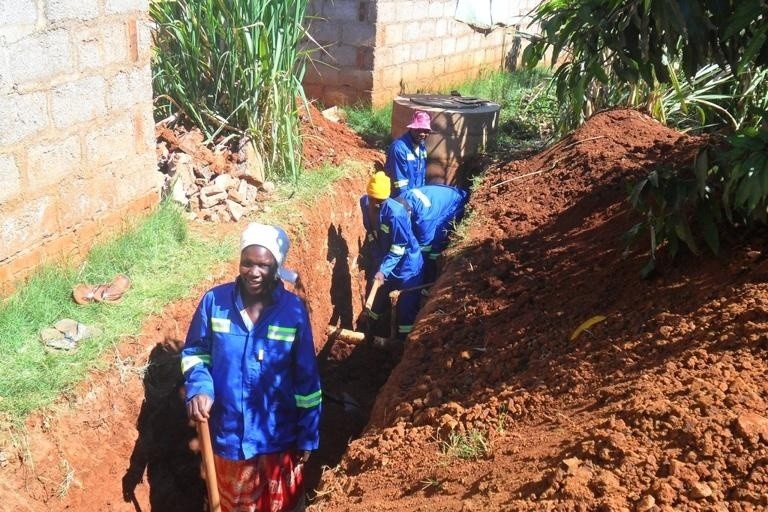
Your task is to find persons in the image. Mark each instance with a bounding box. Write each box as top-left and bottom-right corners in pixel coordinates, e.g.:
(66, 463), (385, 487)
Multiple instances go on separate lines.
(175, 223), (322, 511)
(389, 184), (472, 286)
(385, 112), (432, 194)
(358, 172), (421, 340)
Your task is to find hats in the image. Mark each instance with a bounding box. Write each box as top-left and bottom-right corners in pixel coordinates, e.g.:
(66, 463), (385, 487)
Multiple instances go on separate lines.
(406, 111), (431, 130)
(366, 171), (392, 200)
